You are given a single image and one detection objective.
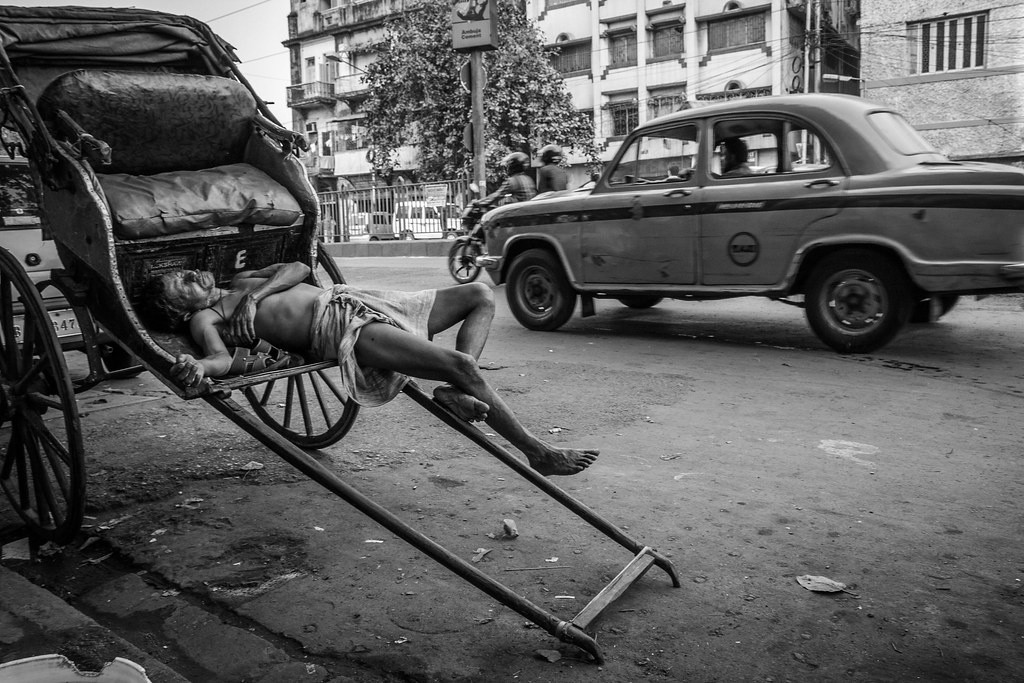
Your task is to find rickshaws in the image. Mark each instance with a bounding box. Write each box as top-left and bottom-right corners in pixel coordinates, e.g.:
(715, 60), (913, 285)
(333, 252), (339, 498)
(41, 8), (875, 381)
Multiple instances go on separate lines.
(0, 4), (682, 666)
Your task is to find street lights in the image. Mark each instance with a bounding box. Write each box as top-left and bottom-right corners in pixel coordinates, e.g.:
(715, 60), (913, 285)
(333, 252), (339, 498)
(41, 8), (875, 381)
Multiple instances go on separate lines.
(324, 52), (381, 212)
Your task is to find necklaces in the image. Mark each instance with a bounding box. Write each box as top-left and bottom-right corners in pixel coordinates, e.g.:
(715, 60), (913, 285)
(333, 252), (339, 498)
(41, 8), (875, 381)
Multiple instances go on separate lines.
(209, 288), (231, 326)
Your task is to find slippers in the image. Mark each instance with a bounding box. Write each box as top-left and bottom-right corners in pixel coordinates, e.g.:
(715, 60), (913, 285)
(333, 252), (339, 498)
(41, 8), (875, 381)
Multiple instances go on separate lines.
(213, 347), (291, 380)
(247, 338), (305, 368)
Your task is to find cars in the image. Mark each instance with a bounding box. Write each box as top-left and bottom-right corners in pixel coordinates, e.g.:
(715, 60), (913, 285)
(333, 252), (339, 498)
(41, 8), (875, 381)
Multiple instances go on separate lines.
(475, 91), (1024, 355)
(339, 200), (467, 239)
(0, 153), (146, 381)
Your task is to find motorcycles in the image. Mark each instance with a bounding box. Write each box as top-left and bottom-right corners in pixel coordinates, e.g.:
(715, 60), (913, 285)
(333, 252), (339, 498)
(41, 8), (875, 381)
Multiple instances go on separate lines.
(448, 182), (498, 285)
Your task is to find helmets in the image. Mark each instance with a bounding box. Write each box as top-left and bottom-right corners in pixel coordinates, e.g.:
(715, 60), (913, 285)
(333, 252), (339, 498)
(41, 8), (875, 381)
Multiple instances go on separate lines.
(538, 145), (563, 165)
(500, 152), (529, 176)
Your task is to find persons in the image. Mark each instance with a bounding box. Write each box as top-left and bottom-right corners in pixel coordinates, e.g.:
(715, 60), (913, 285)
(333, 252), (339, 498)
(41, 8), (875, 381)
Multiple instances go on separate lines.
(720, 138), (753, 175)
(472, 152), (537, 206)
(146, 261), (600, 476)
(537, 144), (569, 191)
